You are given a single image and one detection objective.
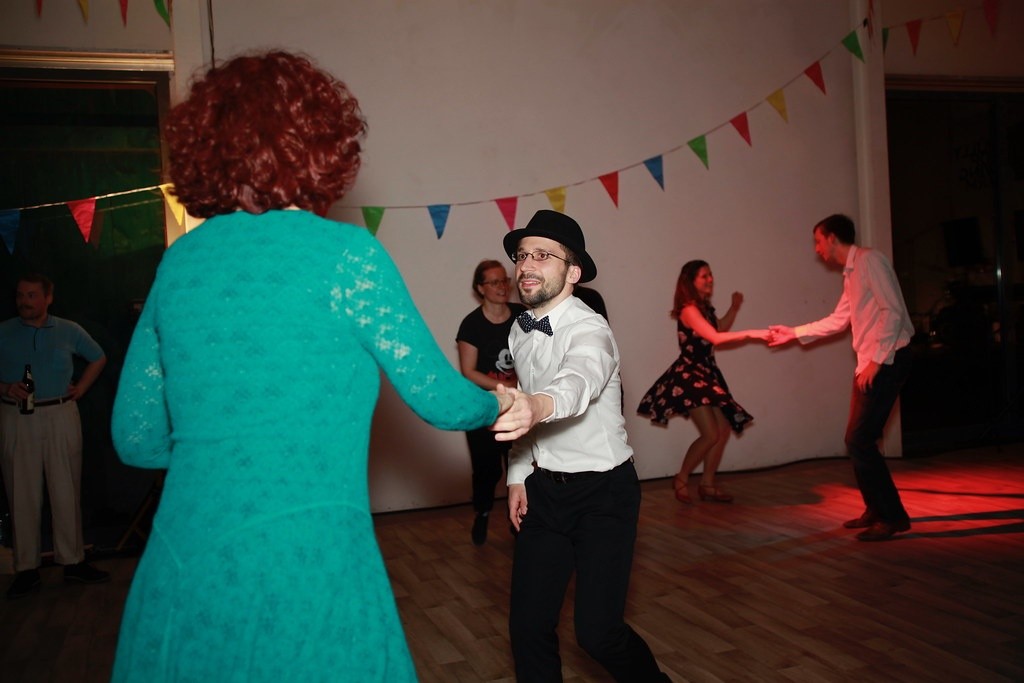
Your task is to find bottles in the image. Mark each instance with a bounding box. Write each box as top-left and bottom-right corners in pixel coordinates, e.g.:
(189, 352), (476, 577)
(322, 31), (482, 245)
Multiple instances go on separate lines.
(20, 364), (34, 414)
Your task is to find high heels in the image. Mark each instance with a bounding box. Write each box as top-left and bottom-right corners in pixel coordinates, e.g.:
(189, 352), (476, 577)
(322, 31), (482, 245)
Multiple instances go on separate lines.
(673, 474), (691, 503)
(698, 484), (732, 502)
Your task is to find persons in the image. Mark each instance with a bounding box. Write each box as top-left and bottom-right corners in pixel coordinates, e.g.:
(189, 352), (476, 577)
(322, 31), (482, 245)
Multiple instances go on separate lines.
(0, 269), (105, 586)
(769, 212), (916, 543)
(635, 257), (775, 504)
(455, 259), (527, 546)
(112, 49), (514, 683)
(490, 208), (674, 683)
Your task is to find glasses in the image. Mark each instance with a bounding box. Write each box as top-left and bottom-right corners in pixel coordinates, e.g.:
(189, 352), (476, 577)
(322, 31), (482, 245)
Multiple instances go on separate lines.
(480, 277), (511, 286)
(511, 250), (575, 268)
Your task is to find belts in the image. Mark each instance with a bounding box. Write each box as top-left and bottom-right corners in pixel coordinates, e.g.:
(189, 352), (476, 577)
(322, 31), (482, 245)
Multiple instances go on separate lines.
(541, 467), (585, 483)
(2, 395), (72, 408)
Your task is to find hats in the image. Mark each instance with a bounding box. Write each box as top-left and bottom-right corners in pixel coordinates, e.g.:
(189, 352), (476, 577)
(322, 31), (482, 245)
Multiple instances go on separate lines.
(503, 209), (597, 283)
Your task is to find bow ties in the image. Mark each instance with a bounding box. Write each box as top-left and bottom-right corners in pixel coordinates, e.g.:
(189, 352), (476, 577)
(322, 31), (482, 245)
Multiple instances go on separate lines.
(517, 311), (553, 337)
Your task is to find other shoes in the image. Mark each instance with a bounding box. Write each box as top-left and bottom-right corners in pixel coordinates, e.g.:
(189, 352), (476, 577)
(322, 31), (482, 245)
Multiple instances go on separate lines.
(63, 560), (109, 583)
(5, 568), (40, 600)
(845, 509), (876, 528)
(471, 514), (490, 546)
(856, 517), (910, 541)
